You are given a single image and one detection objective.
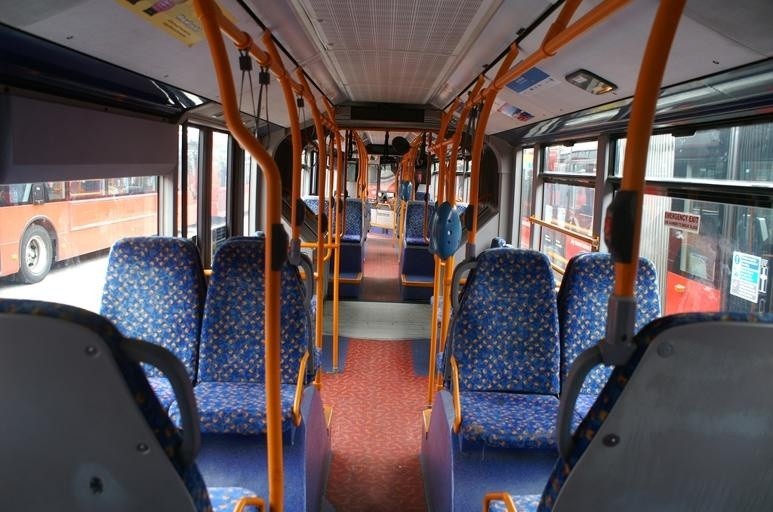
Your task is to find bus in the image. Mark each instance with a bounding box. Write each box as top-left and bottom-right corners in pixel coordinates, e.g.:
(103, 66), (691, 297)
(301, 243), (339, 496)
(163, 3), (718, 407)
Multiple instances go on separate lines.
(0, 126), (220, 283)
(521, 122), (773, 317)
